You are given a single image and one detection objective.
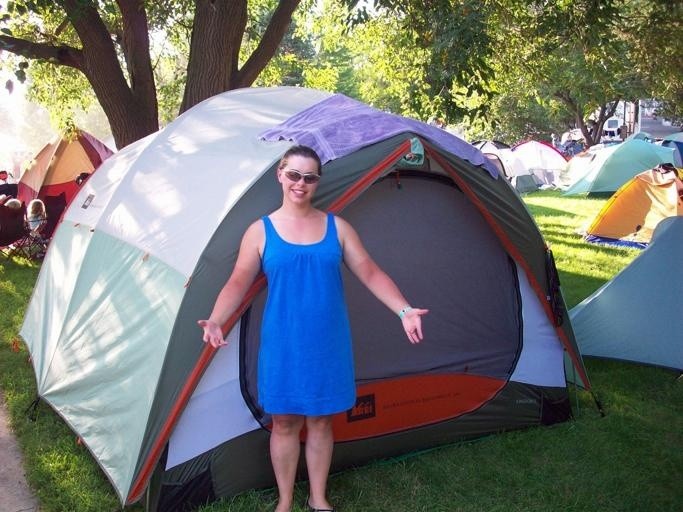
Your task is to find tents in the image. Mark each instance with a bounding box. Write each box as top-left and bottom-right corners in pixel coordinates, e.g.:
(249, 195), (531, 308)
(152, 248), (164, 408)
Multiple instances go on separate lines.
(484, 149), (539, 193)
(568, 214), (683, 370)
(16, 123), (121, 257)
(471, 140), (510, 153)
(556, 131), (683, 200)
(503, 140), (570, 185)
(17, 77), (603, 512)
(582, 164), (683, 250)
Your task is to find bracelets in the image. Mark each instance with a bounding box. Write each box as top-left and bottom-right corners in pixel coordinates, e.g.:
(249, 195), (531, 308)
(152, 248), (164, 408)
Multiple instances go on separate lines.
(398, 305), (413, 320)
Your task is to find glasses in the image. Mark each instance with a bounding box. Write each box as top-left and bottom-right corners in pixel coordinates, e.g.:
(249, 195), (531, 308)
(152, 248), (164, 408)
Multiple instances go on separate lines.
(279, 168), (321, 184)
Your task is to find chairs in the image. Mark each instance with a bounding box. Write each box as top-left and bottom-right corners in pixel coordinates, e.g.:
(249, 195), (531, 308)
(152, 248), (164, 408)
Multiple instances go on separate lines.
(0, 183), (67, 260)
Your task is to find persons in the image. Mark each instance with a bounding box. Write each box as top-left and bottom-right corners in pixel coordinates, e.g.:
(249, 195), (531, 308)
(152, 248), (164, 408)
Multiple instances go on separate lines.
(27, 198), (47, 230)
(0, 169), (21, 211)
(565, 137), (585, 158)
(197, 146), (428, 511)
